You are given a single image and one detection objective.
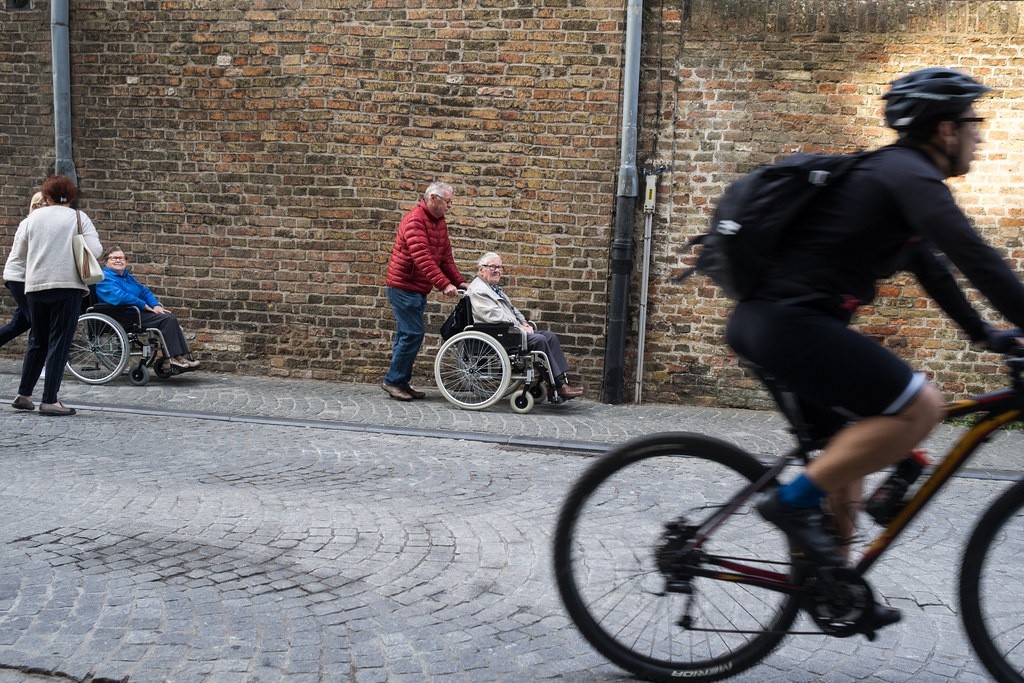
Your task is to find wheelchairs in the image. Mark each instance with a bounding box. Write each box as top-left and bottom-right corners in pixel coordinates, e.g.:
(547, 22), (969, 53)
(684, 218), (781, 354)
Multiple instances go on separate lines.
(66, 282), (201, 387)
(434, 286), (577, 415)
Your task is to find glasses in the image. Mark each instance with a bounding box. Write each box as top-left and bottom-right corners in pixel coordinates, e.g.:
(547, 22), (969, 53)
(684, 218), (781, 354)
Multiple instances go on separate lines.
(481, 264), (505, 272)
(105, 256), (127, 261)
(957, 116), (990, 131)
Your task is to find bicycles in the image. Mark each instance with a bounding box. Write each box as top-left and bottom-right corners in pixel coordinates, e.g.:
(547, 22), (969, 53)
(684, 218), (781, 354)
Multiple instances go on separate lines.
(551, 327), (1024, 683)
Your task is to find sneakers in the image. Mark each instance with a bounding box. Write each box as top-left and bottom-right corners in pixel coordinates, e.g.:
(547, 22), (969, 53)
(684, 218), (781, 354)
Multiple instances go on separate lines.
(800, 596), (904, 630)
(756, 489), (863, 568)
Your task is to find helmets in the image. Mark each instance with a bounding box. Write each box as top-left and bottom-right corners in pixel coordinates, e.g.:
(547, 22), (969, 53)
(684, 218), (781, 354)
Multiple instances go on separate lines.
(881, 68), (992, 133)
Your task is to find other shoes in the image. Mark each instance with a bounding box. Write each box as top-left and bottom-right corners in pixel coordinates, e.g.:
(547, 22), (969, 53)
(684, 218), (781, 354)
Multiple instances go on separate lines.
(548, 383), (584, 402)
(381, 380), (413, 401)
(38, 366), (46, 379)
(162, 357), (189, 374)
(390, 384), (425, 399)
(39, 401), (76, 416)
(12, 395), (36, 410)
(176, 354), (200, 367)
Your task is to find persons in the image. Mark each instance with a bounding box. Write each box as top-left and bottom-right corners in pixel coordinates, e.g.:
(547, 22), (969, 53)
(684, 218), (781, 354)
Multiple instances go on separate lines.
(724, 67), (1024, 634)
(0, 191), (48, 352)
(11, 174), (104, 416)
(97, 246), (201, 370)
(380, 180), (473, 401)
(463, 251), (585, 401)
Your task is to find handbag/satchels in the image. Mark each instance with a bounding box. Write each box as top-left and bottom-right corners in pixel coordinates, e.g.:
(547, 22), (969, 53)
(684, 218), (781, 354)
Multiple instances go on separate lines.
(73, 207), (104, 286)
(440, 298), (466, 344)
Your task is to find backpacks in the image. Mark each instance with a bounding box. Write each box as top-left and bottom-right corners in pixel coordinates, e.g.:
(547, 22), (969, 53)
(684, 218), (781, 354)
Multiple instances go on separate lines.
(698, 144), (932, 302)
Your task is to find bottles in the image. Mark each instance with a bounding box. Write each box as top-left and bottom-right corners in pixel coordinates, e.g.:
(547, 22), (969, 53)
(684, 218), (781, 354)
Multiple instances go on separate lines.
(864, 451), (929, 523)
